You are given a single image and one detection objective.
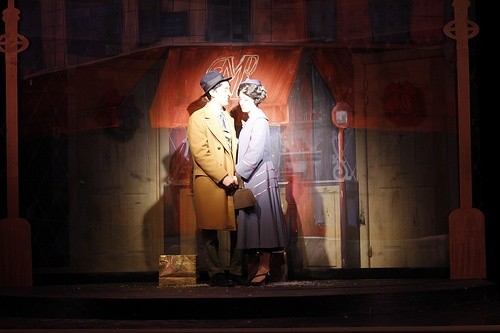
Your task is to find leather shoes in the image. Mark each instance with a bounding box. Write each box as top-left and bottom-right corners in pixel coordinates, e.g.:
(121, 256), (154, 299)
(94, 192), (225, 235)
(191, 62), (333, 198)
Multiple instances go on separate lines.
(212, 272), (250, 287)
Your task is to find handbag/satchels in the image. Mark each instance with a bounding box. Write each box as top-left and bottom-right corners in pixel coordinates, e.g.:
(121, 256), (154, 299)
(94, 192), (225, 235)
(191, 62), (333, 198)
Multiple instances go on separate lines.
(233, 173), (255, 209)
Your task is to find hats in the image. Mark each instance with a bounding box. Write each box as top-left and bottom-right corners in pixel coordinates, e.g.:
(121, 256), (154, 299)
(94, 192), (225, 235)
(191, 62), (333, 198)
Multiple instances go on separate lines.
(200, 70), (232, 97)
(241, 79), (261, 86)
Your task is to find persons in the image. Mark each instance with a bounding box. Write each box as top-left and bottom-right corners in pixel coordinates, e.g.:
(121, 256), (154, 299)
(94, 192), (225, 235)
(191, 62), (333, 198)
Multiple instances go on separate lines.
(234, 79), (286, 286)
(187, 71), (239, 287)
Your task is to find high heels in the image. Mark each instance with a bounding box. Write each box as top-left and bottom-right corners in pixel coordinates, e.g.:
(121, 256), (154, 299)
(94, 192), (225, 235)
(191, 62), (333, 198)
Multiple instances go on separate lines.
(250, 271), (271, 286)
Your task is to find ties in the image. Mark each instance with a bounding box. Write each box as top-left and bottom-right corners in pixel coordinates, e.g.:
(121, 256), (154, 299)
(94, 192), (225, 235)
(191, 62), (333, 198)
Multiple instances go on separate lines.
(220, 112), (231, 153)
